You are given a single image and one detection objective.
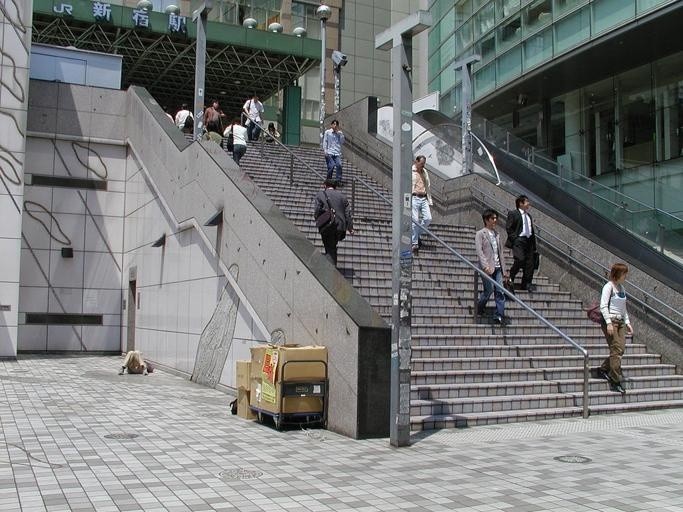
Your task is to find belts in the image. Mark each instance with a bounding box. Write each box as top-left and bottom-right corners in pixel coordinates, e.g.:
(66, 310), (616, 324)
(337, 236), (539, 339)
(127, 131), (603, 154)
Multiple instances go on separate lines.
(412, 193), (427, 198)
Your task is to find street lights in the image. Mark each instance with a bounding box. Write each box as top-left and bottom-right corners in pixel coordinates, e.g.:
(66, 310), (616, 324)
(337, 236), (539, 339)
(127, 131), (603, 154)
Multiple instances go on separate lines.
(315, 0), (328, 148)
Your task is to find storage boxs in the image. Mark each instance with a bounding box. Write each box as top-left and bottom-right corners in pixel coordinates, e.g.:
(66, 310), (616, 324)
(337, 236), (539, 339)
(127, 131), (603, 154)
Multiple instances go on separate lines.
(234, 342), (327, 421)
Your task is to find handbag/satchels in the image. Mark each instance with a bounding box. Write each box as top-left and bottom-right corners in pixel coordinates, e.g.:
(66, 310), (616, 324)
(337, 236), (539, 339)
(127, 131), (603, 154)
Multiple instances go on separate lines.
(227, 133), (233, 151)
(316, 209), (336, 234)
(520, 253), (540, 270)
(503, 280), (515, 302)
(185, 116), (193, 128)
(587, 300), (609, 324)
(241, 112), (248, 127)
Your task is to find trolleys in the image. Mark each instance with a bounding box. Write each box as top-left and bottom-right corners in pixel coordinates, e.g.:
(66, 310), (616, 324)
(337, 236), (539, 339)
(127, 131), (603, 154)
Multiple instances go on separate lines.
(250, 359), (330, 431)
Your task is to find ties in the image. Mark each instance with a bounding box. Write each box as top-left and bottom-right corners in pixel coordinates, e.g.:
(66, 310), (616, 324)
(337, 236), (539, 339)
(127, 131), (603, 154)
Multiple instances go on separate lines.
(525, 214), (529, 238)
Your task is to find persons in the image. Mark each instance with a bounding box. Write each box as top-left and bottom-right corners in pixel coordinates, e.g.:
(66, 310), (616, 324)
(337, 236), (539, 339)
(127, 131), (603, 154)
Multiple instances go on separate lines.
(321, 119), (345, 187)
(411, 154), (433, 251)
(502, 194), (536, 292)
(597, 263), (634, 394)
(222, 116), (249, 166)
(158, 88), (281, 151)
(117, 349), (153, 376)
(474, 208), (511, 325)
(313, 178), (354, 271)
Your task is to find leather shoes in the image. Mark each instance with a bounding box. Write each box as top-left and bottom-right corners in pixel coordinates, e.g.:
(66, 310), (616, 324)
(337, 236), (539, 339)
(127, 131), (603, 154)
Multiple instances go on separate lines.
(477, 303), (507, 325)
(600, 370), (625, 393)
(509, 276), (536, 290)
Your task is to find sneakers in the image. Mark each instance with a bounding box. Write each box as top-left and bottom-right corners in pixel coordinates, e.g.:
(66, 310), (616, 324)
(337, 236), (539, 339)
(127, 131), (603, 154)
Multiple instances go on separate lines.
(411, 238), (422, 250)
